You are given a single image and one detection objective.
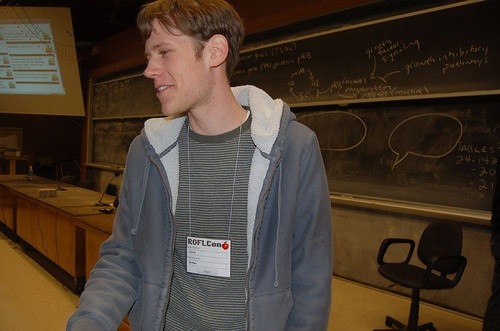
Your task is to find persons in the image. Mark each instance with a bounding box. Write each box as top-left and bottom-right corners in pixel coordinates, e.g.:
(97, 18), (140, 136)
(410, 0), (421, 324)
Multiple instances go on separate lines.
(66, 0), (334, 331)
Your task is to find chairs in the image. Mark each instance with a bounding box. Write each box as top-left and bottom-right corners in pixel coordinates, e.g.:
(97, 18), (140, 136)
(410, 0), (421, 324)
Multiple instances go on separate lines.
(373, 217), (467, 331)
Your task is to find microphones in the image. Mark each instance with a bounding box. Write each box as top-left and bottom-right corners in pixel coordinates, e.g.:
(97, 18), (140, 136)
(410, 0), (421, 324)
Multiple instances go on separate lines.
(96, 172), (119, 206)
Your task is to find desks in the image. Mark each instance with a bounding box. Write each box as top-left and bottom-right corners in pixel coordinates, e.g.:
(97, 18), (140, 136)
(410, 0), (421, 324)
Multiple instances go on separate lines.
(73, 214), (130, 331)
(10, 184), (115, 291)
(0, 174), (76, 233)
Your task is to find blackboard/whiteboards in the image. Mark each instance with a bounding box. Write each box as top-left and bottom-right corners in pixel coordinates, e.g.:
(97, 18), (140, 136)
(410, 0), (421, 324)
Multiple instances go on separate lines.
(85, 1), (500, 229)
